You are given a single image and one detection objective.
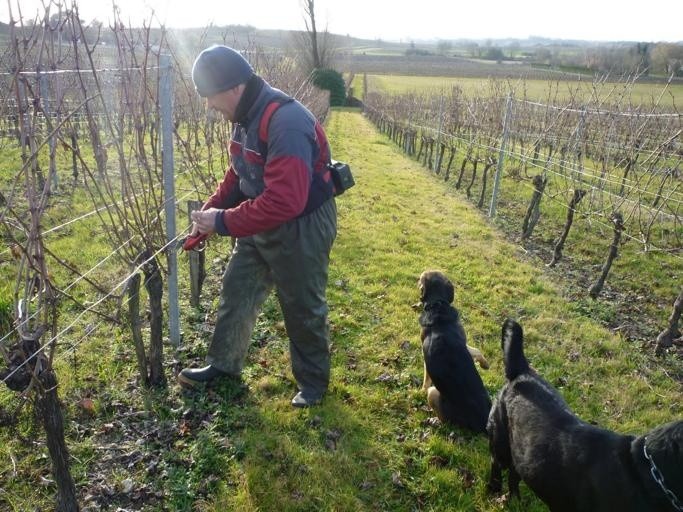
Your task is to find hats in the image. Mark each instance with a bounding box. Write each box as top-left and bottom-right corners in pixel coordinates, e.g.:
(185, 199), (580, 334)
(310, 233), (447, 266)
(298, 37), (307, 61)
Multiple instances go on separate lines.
(193, 48), (254, 96)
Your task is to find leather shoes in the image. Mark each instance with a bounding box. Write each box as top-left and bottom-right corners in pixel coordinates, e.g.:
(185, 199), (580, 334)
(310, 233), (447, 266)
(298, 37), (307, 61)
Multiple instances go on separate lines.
(292, 391), (323, 407)
(178, 365), (242, 386)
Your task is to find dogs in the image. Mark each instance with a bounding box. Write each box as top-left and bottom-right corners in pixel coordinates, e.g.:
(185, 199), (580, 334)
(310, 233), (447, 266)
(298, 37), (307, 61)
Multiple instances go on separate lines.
(484, 315), (683, 512)
(414, 269), (492, 434)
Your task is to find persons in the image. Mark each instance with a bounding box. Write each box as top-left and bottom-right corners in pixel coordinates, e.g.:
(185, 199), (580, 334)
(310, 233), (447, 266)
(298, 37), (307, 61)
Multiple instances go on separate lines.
(175, 46), (338, 406)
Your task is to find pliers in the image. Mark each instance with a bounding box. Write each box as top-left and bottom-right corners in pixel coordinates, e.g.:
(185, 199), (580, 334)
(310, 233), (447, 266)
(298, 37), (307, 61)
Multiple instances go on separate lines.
(176, 233), (209, 255)
